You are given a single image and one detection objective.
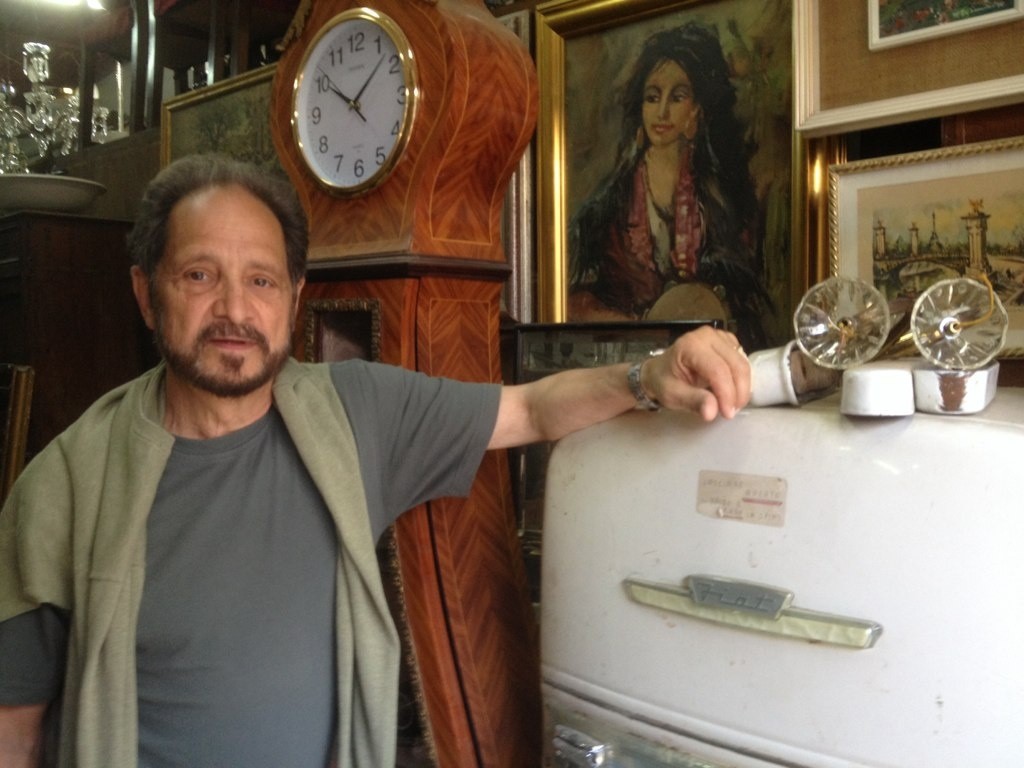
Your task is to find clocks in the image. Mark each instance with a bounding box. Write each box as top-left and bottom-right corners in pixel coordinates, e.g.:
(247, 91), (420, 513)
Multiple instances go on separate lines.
(287, 11), (424, 194)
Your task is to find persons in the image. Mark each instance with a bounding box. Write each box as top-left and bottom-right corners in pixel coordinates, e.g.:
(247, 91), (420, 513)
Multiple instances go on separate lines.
(0, 147), (752, 768)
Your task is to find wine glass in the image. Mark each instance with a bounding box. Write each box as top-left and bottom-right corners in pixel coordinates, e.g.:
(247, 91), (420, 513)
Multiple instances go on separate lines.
(1, 42), (109, 175)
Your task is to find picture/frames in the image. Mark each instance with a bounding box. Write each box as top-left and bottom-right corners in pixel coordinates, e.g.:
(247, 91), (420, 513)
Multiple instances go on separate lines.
(531, 0), (814, 333)
(794, 0), (1024, 145)
(827, 137), (1024, 366)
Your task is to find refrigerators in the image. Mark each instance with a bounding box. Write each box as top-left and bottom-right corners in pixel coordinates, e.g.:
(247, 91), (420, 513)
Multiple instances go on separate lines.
(542, 388), (1023, 765)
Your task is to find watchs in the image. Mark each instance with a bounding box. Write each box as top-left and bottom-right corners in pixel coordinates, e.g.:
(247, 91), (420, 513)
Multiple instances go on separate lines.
(626, 346), (670, 415)
